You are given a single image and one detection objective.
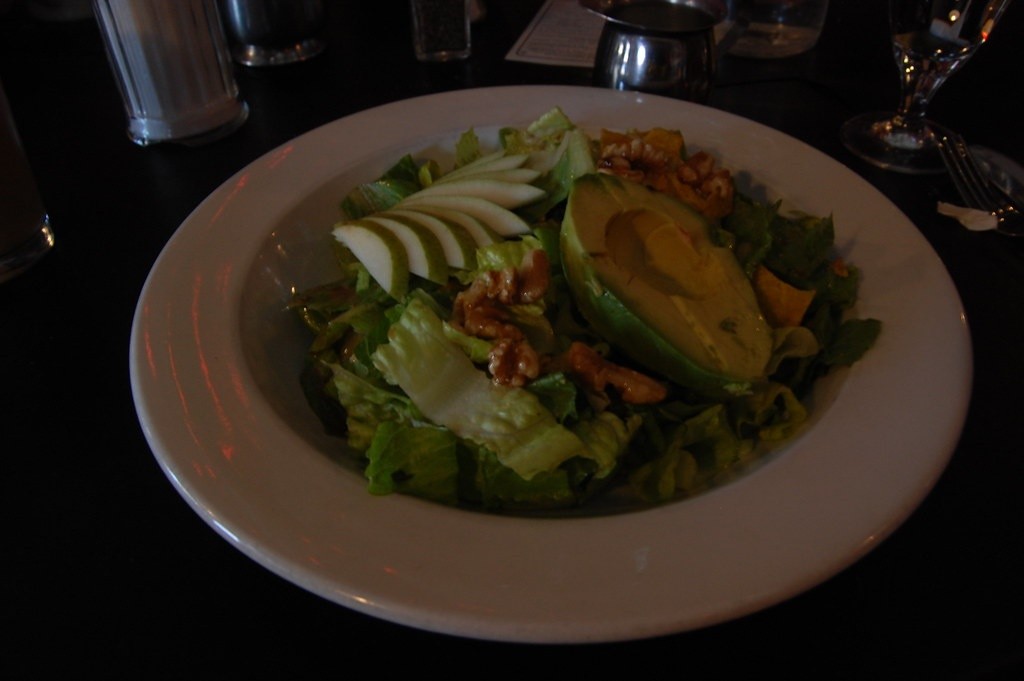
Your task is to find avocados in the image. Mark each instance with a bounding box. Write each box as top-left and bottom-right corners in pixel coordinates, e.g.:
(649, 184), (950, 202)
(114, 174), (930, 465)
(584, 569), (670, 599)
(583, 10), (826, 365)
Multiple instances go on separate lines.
(559, 171), (773, 392)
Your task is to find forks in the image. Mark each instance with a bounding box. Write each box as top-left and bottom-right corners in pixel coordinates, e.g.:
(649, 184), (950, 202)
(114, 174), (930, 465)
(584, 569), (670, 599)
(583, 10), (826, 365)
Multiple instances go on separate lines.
(937, 136), (1024, 237)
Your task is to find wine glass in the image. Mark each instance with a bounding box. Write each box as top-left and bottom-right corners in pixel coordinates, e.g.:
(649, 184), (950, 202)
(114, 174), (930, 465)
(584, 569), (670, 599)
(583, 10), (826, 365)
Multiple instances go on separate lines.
(839, 0), (1011, 176)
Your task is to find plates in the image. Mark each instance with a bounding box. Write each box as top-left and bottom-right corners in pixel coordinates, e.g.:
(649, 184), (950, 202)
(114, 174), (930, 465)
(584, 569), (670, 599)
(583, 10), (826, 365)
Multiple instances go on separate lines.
(128, 85), (973, 645)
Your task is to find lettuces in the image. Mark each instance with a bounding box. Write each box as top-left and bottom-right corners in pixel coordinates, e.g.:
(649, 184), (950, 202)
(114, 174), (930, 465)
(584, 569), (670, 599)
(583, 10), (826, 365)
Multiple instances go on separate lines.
(289, 104), (883, 515)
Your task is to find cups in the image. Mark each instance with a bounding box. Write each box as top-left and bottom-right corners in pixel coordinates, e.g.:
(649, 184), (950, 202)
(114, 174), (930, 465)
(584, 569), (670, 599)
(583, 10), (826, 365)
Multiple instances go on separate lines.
(89, 0), (250, 150)
(227, 0), (327, 66)
(0, 86), (55, 284)
(409, 0), (471, 64)
(576, 0), (728, 106)
